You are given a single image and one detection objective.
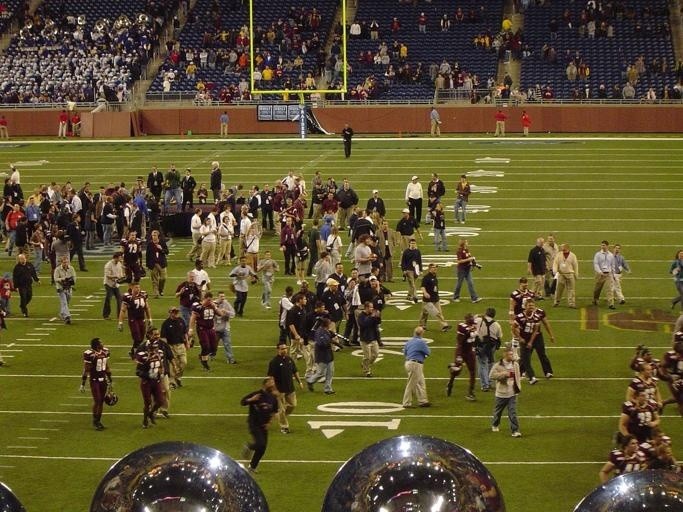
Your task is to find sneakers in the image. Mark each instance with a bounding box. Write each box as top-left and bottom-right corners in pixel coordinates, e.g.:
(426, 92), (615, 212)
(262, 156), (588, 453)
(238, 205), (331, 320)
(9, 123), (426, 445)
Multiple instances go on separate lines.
(261, 301), (266, 306)
(227, 359), (237, 365)
(481, 385), (490, 391)
(148, 413), (156, 424)
(472, 298), (481, 304)
(243, 444), (250, 457)
(159, 408), (169, 418)
(467, 394), (477, 401)
(199, 355), (216, 361)
(281, 428), (289, 433)
(671, 300), (675, 308)
(455, 221), (459, 223)
(93, 419), (104, 430)
(461, 221), (465, 224)
(492, 425), (499, 432)
(446, 382), (452, 396)
(266, 306), (271, 309)
(248, 463), (254, 476)
(568, 305), (577, 309)
(202, 359), (209, 367)
(553, 304), (559, 307)
(453, 298), (460, 302)
(143, 421), (148, 428)
(512, 430), (521, 437)
(546, 372), (552, 378)
(530, 377), (538, 383)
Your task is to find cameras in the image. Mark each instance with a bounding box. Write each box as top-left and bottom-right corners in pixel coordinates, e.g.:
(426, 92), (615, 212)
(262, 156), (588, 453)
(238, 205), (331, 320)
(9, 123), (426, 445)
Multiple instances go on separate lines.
(471, 258), (482, 269)
(62, 277), (74, 286)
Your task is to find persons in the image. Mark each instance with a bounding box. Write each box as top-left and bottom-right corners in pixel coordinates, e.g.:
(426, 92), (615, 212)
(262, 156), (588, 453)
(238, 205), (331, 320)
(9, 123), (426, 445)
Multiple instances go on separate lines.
(474, 308), (504, 393)
(349, 207), (360, 232)
(322, 277), (330, 293)
(425, 173), (445, 227)
(225, 188), (235, 218)
(291, 281), (317, 341)
(433, 204), (449, 253)
(320, 214), (334, 252)
(52, 258), (78, 326)
(599, 435), (650, 487)
(509, 277), (536, 340)
(312, 183), (327, 219)
(282, 184), (296, 205)
(454, 175), (471, 224)
(158, 198), (167, 224)
(1, 2), (163, 106)
(206, 208), (219, 234)
(267, 342), (304, 436)
(365, 190), (386, 219)
(146, 165), (164, 203)
(0, 115), (10, 141)
(277, 287), (294, 348)
(196, 218), (217, 269)
(220, 203), (236, 260)
(446, 313), (481, 401)
(401, 239), (422, 303)
(429, 105), (442, 138)
(325, 228), (344, 267)
(337, 178), (352, 191)
(346, 267), (359, 284)
(286, 294), (308, 362)
(101, 177), (150, 249)
(145, 191), (170, 246)
(329, 186), (339, 196)
(281, 170), (297, 191)
(220, 110), (229, 137)
(237, 210), (252, 264)
(135, 341), (166, 429)
(241, 204), (254, 221)
(297, 172), (306, 196)
(452, 239), (483, 304)
(249, 186), (261, 209)
(160, 308), (190, 390)
(206, 292), (239, 365)
(352, 274), (380, 346)
(610, 244), (632, 305)
(58, 108), (67, 140)
(190, 259), (212, 300)
(347, 268), (360, 284)
(189, 293), (223, 371)
(640, 428), (665, 457)
(624, 364), (660, 408)
(325, 177), (338, 190)
(592, 241), (617, 311)
(175, 272), (202, 349)
(79, 339), (114, 431)
(102, 251), (127, 321)
(13, 254), (42, 317)
(279, 198), (300, 251)
(280, 216), (297, 275)
(373, 212), (380, 226)
(518, 297), (554, 379)
(358, 301), (380, 377)
(405, 176), (424, 225)
(335, 182), (359, 231)
(180, 168), (197, 213)
(542, 236), (560, 298)
(210, 161), (223, 202)
(272, 186), (283, 237)
(71, 113), (80, 135)
(631, 343), (672, 384)
(261, 183), (277, 233)
(619, 385), (662, 441)
(186, 208), (203, 262)
(373, 238), (386, 270)
(241, 377), (280, 475)
(323, 192), (338, 218)
(351, 211), (377, 242)
(402, 327), (433, 409)
(135, 327), (181, 420)
(521, 110), (530, 137)
(552, 244), (579, 309)
(229, 183), (244, 194)
(345, 234), (359, 266)
(243, 222), (261, 285)
(293, 179), (301, 200)
(658, 332), (683, 413)
(361, 210), (374, 225)
(316, 279), (350, 354)
(307, 171), (323, 219)
(162, 0), (683, 105)
(229, 257), (259, 318)
(670, 251), (683, 310)
(527, 238), (549, 302)
(512, 300), (554, 386)
(118, 282), (153, 360)
(341, 122), (353, 159)
(306, 220), (322, 277)
(145, 230), (170, 300)
(256, 251), (280, 310)
(314, 252), (333, 301)
(368, 235), (377, 269)
(375, 221), (397, 284)
(197, 182), (208, 204)
(163, 164), (182, 217)
(488, 350), (523, 439)
(354, 233), (377, 278)
(673, 313), (683, 361)
(306, 318), (338, 395)
(369, 266), (380, 281)
(360, 275), (385, 349)
(214, 216), (234, 267)
(328, 265), (347, 294)
(293, 193), (309, 233)
(0, 161), (100, 268)
(304, 301), (331, 384)
(647, 443), (678, 470)
(418, 263), (453, 334)
(397, 209), (422, 267)
(0, 274), (19, 317)
(294, 223), (312, 287)
(494, 109), (508, 138)
(119, 231), (143, 284)
(343, 279), (360, 347)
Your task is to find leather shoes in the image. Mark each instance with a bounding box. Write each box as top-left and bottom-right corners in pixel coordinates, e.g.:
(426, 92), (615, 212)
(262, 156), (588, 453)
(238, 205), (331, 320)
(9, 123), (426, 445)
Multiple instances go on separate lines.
(593, 300), (599, 304)
(65, 317), (71, 323)
(308, 381), (314, 392)
(24, 312), (28, 316)
(414, 298), (418, 303)
(609, 305), (615, 309)
(420, 403), (432, 407)
(407, 295), (412, 300)
(367, 373), (372, 377)
(441, 326), (452, 332)
(404, 406), (416, 408)
(324, 391), (335, 395)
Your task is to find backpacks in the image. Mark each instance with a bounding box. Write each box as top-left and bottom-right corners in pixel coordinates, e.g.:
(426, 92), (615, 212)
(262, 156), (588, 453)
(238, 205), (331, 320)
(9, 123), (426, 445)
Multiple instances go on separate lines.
(480, 316), (500, 357)
(325, 235), (338, 254)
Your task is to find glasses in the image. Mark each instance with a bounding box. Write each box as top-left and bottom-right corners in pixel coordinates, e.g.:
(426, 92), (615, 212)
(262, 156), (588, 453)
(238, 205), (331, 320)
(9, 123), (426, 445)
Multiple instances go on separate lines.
(431, 267), (436, 268)
(169, 309), (179, 313)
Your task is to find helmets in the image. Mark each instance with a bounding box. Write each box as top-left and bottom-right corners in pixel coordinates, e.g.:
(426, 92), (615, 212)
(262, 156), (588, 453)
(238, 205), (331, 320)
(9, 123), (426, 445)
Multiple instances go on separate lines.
(449, 364), (462, 377)
(140, 266), (146, 277)
(105, 393), (118, 406)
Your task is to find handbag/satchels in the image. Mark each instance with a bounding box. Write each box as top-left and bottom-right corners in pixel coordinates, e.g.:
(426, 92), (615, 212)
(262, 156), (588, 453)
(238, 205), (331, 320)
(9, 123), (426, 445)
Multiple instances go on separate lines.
(229, 282), (235, 293)
(198, 235), (206, 244)
(475, 336), (481, 355)
(244, 237), (256, 252)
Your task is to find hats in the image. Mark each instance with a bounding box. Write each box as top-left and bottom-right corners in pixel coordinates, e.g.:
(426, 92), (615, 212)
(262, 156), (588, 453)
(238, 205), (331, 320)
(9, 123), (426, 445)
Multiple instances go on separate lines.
(0, 278), (17, 292)
(186, 168), (192, 172)
(369, 276), (377, 280)
(401, 208), (410, 213)
(412, 176), (418, 180)
(373, 189), (378, 193)
(326, 278), (340, 286)
(347, 277), (357, 284)
(137, 176), (145, 182)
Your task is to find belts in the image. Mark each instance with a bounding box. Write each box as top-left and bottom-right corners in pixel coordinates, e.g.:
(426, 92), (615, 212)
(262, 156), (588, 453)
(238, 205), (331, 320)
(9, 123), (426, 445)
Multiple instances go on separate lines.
(564, 272), (576, 274)
(405, 358), (424, 364)
(603, 272), (609, 274)
